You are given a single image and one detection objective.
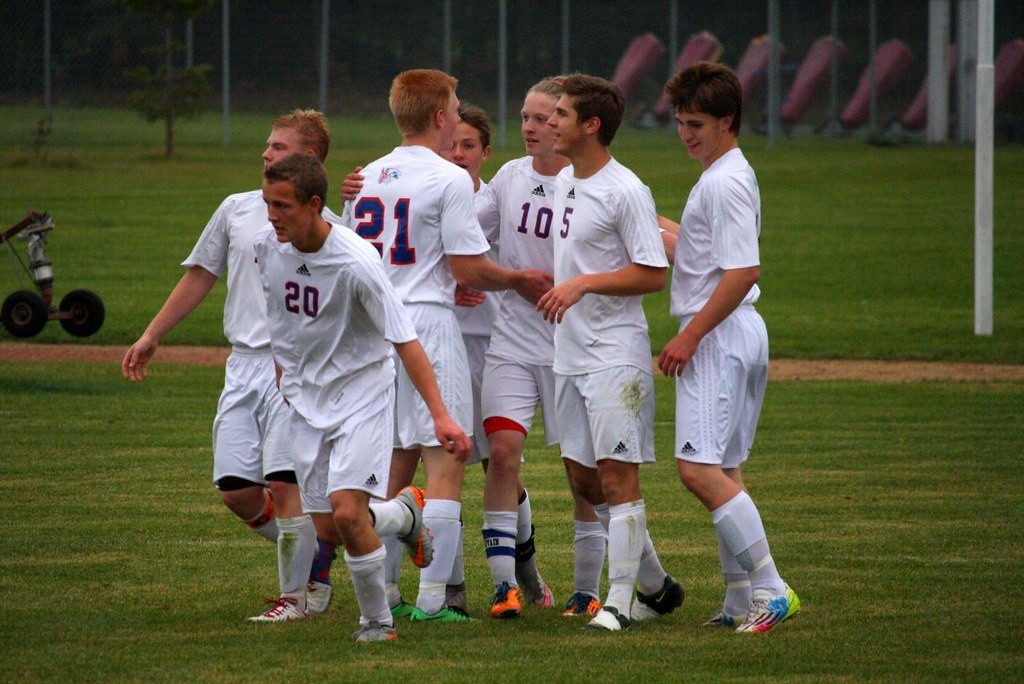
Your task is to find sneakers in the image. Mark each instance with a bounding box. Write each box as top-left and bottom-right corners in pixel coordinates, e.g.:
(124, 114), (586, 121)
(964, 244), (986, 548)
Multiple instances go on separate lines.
(390, 600), (413, 619)
(351, 622), (398, 643)
(409, 607), (482, 624)
(631, 575), (688, 622)
(489, 582), (522, 619)
(396, 485), (435, 569)
(562, 594), (602, 617)
(446, 591), (470, 607)
(587, 602), (632, 631)
(248, 600), (311, 622)
(701, 584), (802, 634)
(305, 538), (337, 615)
(528, 583), (554, 609)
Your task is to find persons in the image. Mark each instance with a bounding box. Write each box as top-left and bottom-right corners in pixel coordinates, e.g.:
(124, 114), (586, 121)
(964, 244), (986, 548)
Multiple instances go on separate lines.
(341, 74), (683, 620)
(338, 69), (562, 625)
(656, 59), (799, 635)
(434, 102), (557, 619)
(251, 152), (475, 644)
(121, 106), (356, 624)
(534, 71), (684, 635)
